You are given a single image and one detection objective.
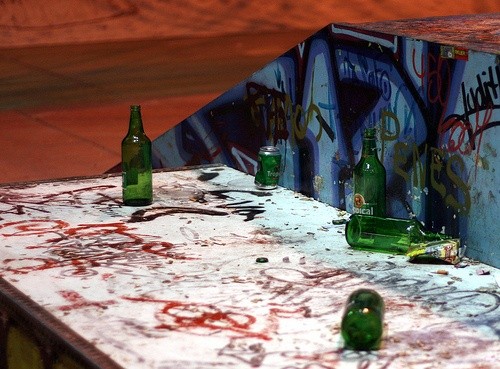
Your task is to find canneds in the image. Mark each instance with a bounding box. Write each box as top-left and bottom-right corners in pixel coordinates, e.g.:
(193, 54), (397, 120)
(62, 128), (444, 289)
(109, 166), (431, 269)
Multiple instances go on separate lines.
(408, 235), (461, 265)
(253, 146), (281, 190)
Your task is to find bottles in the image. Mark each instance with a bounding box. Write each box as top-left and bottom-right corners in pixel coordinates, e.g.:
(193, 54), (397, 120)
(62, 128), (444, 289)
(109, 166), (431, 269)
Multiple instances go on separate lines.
(345, 212), (453, 254)
(352, 128), (388, 218)
(120, 104), (153, 205)
(342, 287), (384, 347)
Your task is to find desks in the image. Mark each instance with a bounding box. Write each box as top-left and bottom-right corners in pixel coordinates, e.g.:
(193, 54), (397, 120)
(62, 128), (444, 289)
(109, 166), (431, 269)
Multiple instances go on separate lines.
(0, 165), (500, 369)
